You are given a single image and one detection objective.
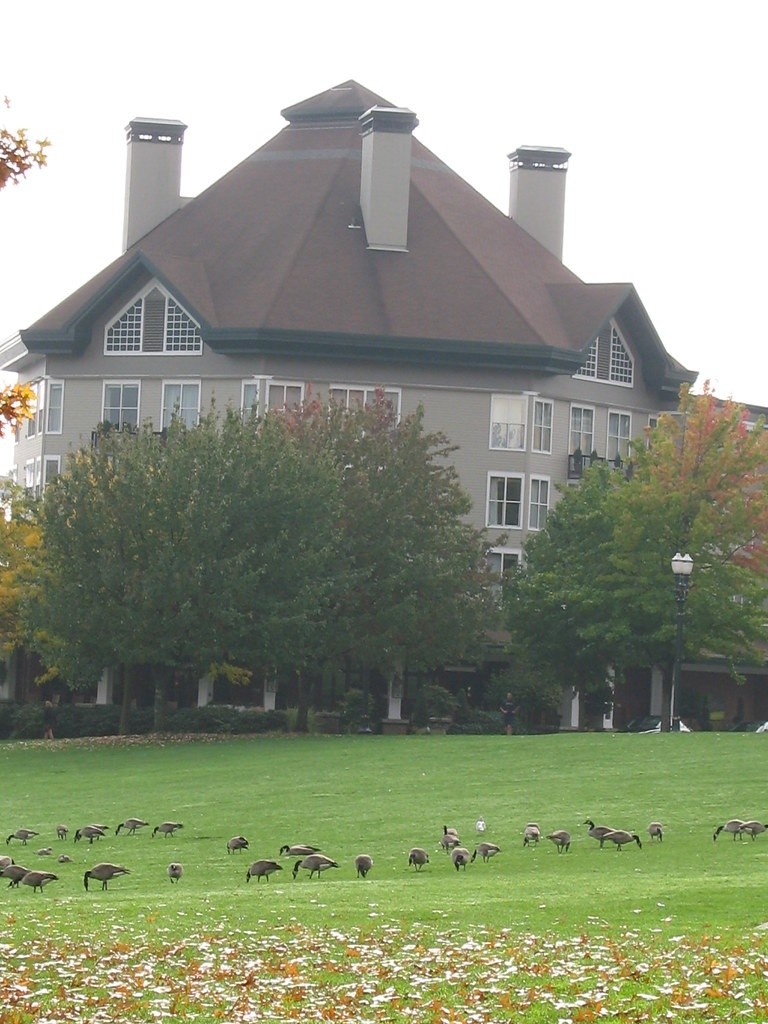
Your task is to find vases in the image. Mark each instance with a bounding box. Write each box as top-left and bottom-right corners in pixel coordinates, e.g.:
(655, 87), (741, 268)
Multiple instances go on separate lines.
(382, 719), (409, 735)
(316, 712), (342, 734)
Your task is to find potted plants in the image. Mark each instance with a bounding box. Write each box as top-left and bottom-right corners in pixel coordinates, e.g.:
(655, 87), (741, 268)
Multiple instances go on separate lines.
(419, 684), (461, 734)
(342, 688), (375, 733)
(704, 694), (726, 720)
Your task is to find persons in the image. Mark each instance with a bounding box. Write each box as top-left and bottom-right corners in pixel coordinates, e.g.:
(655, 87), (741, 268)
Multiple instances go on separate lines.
(501, 693), (518, 735)
(44, 700), (54, 739)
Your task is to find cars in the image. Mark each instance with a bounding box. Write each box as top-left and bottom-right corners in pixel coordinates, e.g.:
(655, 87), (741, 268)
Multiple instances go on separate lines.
(732, 720), (768, 733)
(613, 713), (692, 733)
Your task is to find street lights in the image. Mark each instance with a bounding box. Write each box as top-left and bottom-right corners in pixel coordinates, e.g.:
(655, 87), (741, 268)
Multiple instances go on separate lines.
(670, 551), (694, 731)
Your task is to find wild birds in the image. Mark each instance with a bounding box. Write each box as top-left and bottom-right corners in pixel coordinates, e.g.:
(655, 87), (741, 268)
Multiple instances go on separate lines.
(0, 817), (184, 892)
(646, 821), (662, 841)
(582, 819), (642, 851)
(246, 860), (283, 883)
(546, 830), (571, 854)
(442, 816), (502, 872)
(523, 822), (541, 847)
(409, 848), (429, 871)
(279, 844), (341, 880)
(355, 854), (373, 878)
(713, 820), (768, 841)
(227, 836), (251, 854)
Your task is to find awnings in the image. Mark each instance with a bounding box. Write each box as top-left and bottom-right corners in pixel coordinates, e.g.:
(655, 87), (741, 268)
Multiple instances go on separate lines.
(679, 638), (768, 676)
